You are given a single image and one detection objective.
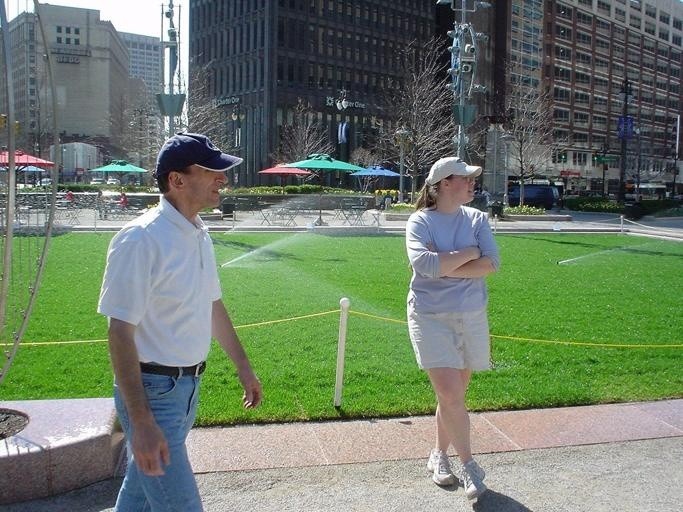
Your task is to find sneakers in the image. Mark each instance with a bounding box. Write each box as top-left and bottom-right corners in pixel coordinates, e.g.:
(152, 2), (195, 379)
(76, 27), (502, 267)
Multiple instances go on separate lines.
(426, 448), (455, 487)
(460, 460), (487, 500)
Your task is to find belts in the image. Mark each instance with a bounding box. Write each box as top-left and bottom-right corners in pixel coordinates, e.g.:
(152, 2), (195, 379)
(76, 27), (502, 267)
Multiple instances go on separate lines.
(140, 360), (206, 377)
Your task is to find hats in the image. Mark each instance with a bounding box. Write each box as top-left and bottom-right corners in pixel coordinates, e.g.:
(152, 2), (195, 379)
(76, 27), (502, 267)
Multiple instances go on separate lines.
(156, 132), (243, 172)
(426, 156), (484, 184)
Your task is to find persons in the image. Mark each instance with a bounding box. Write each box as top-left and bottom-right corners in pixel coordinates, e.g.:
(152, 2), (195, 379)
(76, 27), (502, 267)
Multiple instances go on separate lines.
(376, 190), (398, 208)
(482, 186), (492, 202)
(474, 183), (482, 195)
(405, 155), (501, 501)
(120, 193), (127, 209)
(97, 129), (263, 512)
(65, 190), (73, 200)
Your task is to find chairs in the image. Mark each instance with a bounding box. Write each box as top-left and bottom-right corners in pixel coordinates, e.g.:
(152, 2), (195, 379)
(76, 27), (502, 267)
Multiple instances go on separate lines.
(256, 196), (384, 227)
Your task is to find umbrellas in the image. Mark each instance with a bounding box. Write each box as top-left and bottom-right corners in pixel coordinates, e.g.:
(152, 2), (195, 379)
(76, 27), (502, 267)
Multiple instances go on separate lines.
(88, 159), (149, 192)
(258, 162), (313, 194)
(350, 163), (401, 188)
(0, 150), (55, 221)
(0, 166), (46, 193)
(281, 152), (366, 225)
